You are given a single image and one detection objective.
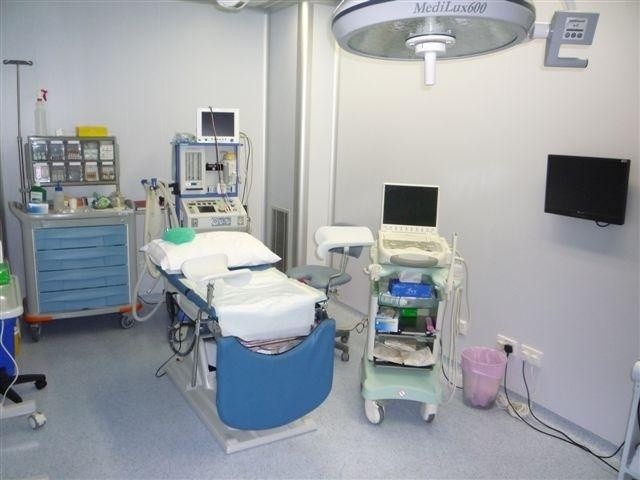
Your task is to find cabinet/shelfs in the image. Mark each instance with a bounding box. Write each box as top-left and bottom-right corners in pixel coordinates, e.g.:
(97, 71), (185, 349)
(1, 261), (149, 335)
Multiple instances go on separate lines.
(361, 231), (460, 423)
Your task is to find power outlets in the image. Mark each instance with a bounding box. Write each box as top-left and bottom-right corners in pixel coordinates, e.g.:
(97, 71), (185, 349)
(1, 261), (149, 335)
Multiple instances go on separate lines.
(496, 334), (543, 367)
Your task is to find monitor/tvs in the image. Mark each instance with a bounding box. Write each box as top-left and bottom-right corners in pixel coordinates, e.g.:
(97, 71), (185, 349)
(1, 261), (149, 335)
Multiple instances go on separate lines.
(544, 154), (631, 225)
(196, 108), (240, 144)
(381, 183), (440, 228)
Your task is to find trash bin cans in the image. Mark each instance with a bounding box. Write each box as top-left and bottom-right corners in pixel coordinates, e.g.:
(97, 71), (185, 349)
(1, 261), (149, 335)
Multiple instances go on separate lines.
(461, 346), (508, 410)
(0, 275), (24, 377)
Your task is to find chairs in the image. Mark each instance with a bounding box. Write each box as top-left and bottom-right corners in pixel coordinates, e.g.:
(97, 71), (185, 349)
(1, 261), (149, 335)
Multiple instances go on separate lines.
(285, 225), (363, 361)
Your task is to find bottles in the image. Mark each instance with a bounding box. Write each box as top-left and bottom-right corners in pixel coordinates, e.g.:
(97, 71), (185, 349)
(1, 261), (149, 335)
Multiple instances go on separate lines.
(52, 184), (65, 215)
(34, 97), (47, 134)
(29, 183), (46, 204)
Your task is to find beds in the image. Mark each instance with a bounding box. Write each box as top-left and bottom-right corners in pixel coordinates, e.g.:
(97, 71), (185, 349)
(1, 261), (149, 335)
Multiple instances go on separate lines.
(157, 233), (333, 442)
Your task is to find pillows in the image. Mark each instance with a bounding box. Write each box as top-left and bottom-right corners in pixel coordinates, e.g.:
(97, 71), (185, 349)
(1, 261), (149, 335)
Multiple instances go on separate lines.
(139, 231), (283, 274)
